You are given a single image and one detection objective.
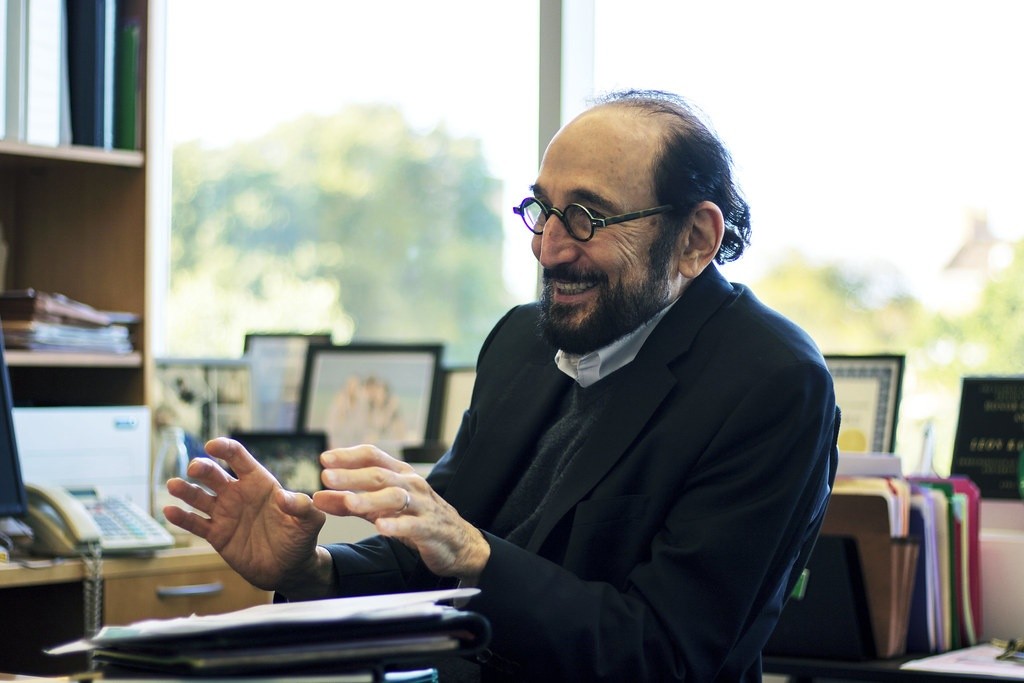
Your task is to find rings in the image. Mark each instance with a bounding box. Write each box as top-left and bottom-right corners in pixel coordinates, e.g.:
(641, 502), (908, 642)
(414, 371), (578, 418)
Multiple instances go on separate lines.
(395, 488), (411, 514)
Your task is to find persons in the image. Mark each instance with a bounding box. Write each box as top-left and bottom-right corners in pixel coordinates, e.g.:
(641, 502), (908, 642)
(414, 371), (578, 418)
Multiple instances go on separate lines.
(161, 88), (841, 683)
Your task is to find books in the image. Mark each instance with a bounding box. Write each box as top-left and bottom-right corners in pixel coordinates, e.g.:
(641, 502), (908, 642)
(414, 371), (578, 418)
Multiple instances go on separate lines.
(92, 612), (490, 683)
(0, 288), (138, 356)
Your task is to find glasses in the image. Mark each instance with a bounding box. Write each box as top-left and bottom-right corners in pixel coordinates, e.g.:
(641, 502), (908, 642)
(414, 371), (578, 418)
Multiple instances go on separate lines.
(512, 196), (676, 242)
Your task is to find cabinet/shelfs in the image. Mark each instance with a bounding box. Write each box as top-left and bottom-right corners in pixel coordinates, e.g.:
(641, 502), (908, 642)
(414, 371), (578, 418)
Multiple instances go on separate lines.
(0, 0), (274, 629)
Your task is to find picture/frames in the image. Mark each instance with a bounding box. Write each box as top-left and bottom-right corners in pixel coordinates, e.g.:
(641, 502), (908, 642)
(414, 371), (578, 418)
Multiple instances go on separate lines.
(822, 354), (904, 454)
(295, 342), (443, 447)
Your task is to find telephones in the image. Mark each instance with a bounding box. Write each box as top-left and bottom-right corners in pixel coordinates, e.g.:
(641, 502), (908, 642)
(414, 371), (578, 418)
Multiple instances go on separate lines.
(22, 483), (177, 557)
